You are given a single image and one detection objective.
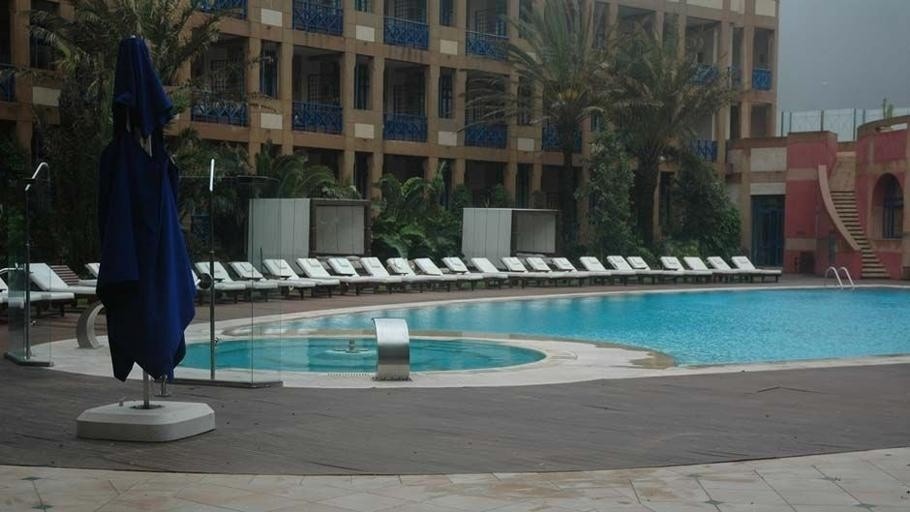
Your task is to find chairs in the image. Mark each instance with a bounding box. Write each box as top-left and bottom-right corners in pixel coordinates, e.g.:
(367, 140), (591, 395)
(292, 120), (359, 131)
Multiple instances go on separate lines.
(1, 255), (782, 323)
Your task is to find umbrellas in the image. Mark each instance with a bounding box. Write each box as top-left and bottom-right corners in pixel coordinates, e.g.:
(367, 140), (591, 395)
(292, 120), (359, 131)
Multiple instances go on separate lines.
(97, 32), (196, 406)
